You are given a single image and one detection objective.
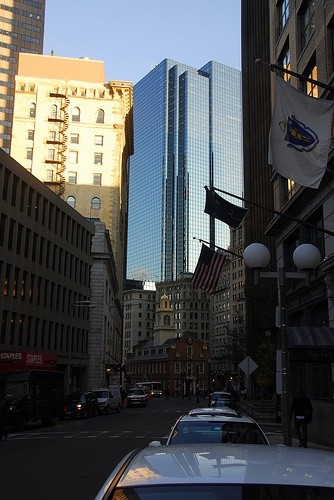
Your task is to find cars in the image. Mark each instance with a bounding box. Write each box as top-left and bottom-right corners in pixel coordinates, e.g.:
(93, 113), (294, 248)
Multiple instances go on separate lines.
(64, 391), (101, 419)
(186, 406), (239, 417)
(110, 389), (128, 409)
(88, 388), (122, 415)
(210, 392), (234, 407)
(211, 398), (242, 418)
(93, 442), (334, 500)
(126, 387), (148, 408)
(147, 413), (285, 446)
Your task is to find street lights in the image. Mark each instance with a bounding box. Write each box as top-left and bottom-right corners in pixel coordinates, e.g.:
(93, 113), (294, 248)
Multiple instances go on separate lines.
(196, 362), (200, 403)
(66, 300), (97, 396)
(187, 366), (191, 401)
(241, 242), (322, 445)
(207, 358), (211, 407)
(105, 363), (122, 393)
(180, 369), (187, 400)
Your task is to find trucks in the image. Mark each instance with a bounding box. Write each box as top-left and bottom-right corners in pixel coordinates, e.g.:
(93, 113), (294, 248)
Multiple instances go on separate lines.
(0, 367), (65, 441)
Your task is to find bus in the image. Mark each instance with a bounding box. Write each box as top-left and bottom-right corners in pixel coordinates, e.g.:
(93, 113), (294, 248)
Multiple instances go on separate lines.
(135, 382), (163, 398)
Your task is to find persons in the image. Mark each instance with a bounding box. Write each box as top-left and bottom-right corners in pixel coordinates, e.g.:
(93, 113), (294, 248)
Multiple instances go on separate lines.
(290, 387), (307, 448)
(239, 382), (248, 400)
(120, 388), (125, 408)
(227, 382), (238, 398)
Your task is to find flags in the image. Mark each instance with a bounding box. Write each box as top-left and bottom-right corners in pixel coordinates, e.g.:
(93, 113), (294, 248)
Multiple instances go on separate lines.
(267, 72), (334, 189)
(204, 189), (249, 230)
(189, 243), (227, 295)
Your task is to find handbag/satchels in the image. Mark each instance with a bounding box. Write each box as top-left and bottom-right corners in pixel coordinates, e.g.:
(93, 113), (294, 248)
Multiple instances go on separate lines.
(304, 409), (312, 423)
